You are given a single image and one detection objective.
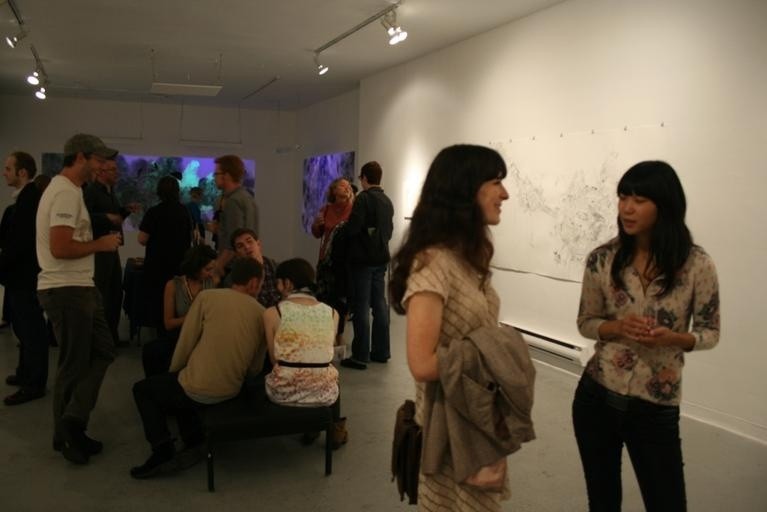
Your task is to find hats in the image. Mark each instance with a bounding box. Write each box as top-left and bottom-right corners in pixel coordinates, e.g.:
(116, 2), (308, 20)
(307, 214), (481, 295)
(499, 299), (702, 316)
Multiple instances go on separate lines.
(64, 132), (118, 162)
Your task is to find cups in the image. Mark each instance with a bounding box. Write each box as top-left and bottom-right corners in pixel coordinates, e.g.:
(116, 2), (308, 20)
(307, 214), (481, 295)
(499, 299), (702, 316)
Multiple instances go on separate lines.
(109, 231), (119, 243)
(639, 298), (657, 334)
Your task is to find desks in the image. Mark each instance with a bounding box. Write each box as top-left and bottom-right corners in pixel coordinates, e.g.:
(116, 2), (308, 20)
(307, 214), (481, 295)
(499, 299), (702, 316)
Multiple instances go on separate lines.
(120, 252), (220, 344)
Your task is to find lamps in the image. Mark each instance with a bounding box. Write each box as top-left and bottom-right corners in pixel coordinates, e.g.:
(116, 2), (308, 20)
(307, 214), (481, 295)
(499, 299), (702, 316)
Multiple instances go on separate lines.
(4, 29), (29, 49)
(24, 61), (51, 102)
(312, 5), (407, 80)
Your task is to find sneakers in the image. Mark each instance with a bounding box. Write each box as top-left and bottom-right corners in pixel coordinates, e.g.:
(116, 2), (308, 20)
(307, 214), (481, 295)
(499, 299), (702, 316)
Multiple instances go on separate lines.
(341, 357), (367, 371)
(174, 443), (206, 470)
(129, 447), (174, 480)
(369, 351), (389, 363)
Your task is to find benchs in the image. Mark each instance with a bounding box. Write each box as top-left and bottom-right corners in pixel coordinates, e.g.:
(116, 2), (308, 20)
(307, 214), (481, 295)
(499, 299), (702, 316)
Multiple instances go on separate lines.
(177, 390), (342, 495)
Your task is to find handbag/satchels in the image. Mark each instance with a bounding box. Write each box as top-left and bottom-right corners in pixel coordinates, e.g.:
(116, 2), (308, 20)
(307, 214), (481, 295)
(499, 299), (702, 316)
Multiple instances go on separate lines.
(391, 398), (422, 504)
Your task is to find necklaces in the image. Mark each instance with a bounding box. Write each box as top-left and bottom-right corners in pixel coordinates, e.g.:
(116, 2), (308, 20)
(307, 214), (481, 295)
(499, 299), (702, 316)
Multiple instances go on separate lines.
(464, 262), (481, 280)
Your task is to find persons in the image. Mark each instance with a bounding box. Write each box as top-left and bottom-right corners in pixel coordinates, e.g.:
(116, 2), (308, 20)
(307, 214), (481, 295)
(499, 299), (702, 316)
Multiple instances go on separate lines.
(570, 159), (722, 512)
(82, 148), (260, 347)
(36, 135), (121, 465)
(385, 141), (515, 511)
(0, 151), (52, 405)
(312, 161), (394, 370)
(129, 228), (348, 481)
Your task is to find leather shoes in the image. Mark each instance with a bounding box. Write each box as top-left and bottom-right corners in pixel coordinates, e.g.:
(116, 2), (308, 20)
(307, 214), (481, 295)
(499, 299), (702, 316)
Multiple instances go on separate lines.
(4, 373), (46, 404)
(53, 433), (103, 464)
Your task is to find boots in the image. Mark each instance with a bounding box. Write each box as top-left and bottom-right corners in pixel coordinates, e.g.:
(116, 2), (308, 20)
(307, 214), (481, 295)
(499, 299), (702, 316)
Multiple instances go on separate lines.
(304, 416), (349, 450)
